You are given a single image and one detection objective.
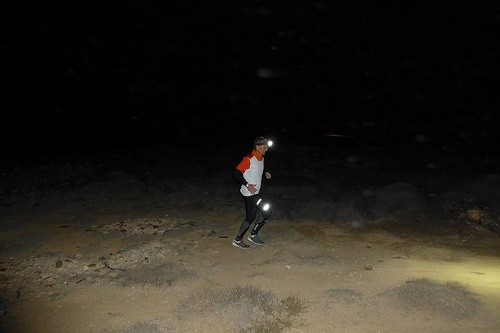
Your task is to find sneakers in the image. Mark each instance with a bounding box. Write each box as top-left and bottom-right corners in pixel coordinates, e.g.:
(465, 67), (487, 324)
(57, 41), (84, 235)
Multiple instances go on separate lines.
(247, 232), (265, 246)
(232, 238), (251, 250)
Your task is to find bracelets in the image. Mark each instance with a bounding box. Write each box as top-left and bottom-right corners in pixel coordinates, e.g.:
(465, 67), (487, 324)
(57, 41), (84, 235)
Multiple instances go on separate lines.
(246, 183), (249, 187)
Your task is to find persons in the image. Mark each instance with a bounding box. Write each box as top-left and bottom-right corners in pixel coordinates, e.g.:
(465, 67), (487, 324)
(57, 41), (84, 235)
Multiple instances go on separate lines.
(231, 135), (273, 250)
